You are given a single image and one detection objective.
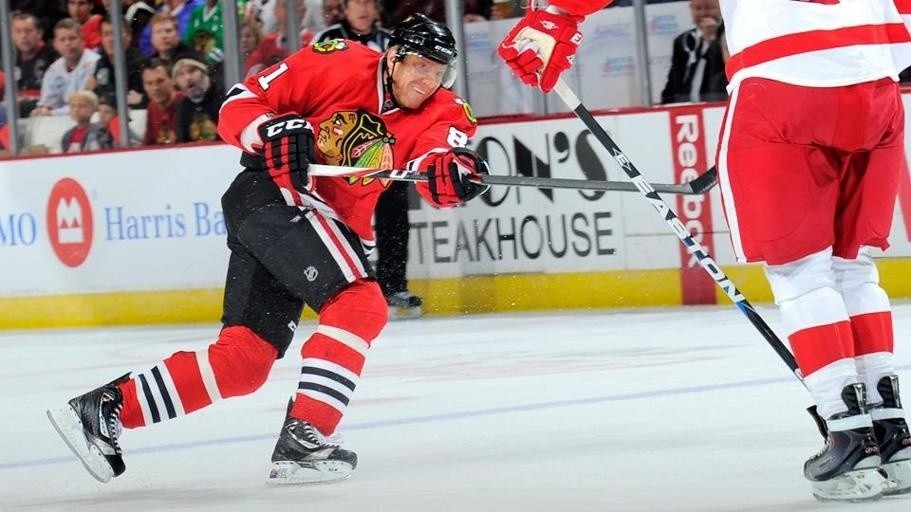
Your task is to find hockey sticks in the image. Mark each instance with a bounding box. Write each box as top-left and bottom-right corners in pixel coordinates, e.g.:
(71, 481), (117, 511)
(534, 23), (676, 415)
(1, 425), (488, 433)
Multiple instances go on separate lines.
(241, 150), (718, 195)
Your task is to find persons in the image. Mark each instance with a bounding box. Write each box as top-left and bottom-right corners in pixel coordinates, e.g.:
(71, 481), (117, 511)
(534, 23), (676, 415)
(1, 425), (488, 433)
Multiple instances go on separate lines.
(309, 0), (423, 307)
(69, 13), (491, 477)
(2, 1), (528, 159)
(661, 0), (729, 105)
(498, 0), (911, 483)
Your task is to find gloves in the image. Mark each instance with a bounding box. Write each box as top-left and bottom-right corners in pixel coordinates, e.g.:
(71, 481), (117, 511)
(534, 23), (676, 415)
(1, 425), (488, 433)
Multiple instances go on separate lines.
(496, 0), (585, 94)
(257, 111), (325, 194)
(416, 147), (492, 208)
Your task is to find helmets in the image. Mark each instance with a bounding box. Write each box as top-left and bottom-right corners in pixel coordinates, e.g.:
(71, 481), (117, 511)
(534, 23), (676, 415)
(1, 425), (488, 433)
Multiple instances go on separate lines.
(384, 13), (458, 90)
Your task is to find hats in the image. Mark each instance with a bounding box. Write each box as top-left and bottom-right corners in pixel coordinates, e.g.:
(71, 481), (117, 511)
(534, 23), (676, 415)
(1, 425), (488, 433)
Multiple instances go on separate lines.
(166, 43), (212, 80)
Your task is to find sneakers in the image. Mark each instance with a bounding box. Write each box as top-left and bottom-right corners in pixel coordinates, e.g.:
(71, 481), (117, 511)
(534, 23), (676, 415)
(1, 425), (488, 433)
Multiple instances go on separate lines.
(271, 395), (357, 470)
(68, 371), (133, 477)
(804, 374), (911, 481)
(383, 291), (422, 308)
